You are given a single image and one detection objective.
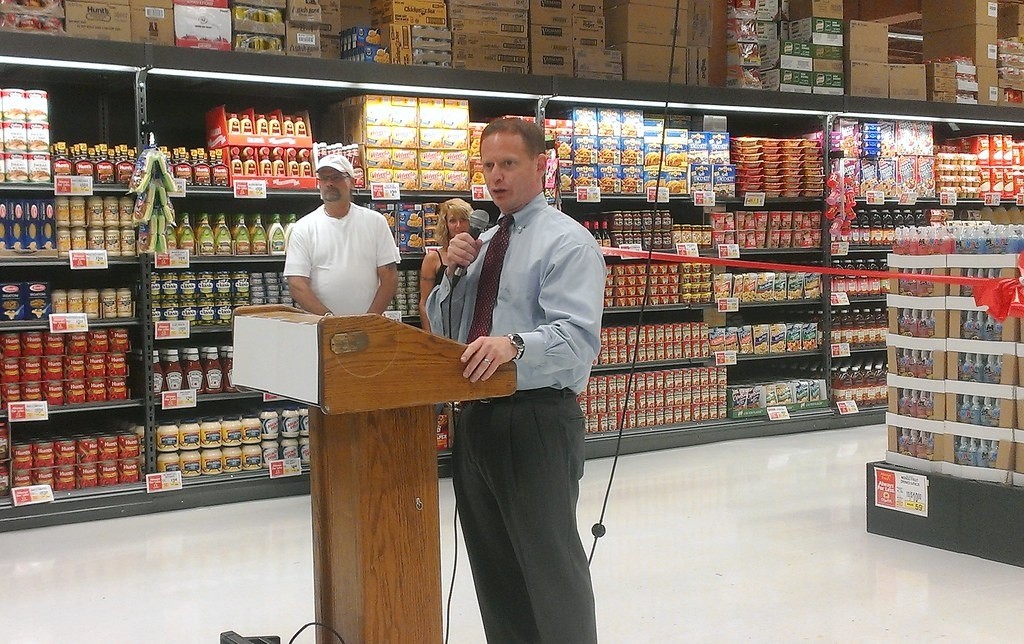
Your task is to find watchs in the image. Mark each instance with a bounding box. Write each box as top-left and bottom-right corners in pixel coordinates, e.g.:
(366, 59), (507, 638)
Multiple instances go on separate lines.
(504, 333), (525, 360)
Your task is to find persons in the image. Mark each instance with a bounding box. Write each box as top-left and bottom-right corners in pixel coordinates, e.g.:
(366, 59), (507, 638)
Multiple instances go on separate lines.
(435, 117), (608, 644)
(418, 197), (475, 333)
(283, 154), (401, 316)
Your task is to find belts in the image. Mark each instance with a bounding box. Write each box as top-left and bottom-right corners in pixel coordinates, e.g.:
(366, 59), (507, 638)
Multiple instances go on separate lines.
(479, 387), (575, 406)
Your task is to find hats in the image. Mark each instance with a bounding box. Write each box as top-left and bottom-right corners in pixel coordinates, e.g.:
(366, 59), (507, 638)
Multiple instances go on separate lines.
(316, 154), (354, 179)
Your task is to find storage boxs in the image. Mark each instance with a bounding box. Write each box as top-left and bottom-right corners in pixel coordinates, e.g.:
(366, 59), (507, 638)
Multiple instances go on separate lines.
(0, 0), (1024, 419)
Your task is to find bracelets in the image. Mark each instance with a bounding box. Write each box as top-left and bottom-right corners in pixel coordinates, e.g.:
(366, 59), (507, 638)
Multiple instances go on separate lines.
(324, 310), (334, 318)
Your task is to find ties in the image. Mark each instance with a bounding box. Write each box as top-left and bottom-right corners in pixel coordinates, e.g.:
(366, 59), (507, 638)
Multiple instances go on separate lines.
(464, 213), (513, 345)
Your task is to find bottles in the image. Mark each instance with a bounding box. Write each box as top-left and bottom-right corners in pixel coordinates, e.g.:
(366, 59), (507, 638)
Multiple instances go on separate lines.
(780, 308), (825, 349)
(962, 311), (1002, 341)
(897, 390), (936, 460)
(955, 351), (1001, 467)
(957, 222), (1024, 254)
(51, 142), (137, 185)
(830, 308), (888, 347)
(897, 349), (933, 379)
(813, 260), (890, 297)
(832, 355), (888, 406)
(583, 221), (611, 247)
(226, 114), (312, 176)
(852, 210), (927, 245)
(899, 308), (935, 338)
(318, 142), (364, 188)
(900, 268), (934, 297)
(893, 227), (956, 255)
(160, 146), (229, 186)
(169, 212), (298, 257)
(782, 358), (823, 380)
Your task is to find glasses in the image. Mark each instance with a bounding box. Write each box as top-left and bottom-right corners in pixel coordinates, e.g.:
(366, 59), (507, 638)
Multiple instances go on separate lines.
(317, 172), (347, 184)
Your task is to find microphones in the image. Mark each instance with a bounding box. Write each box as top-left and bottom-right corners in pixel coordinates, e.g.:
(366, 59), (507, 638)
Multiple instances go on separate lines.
(451, 210), (489, 288)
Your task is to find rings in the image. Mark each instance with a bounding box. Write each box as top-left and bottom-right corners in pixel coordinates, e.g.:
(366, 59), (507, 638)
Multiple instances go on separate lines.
(484, 358), (491, 364)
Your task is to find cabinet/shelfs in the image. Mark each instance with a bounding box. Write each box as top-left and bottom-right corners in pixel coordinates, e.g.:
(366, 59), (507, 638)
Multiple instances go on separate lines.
(513, 75), (835, 461)
(0, 33), (154, 535)
(796, 94), (1024, 427)
(103, 43), (553, 511)
